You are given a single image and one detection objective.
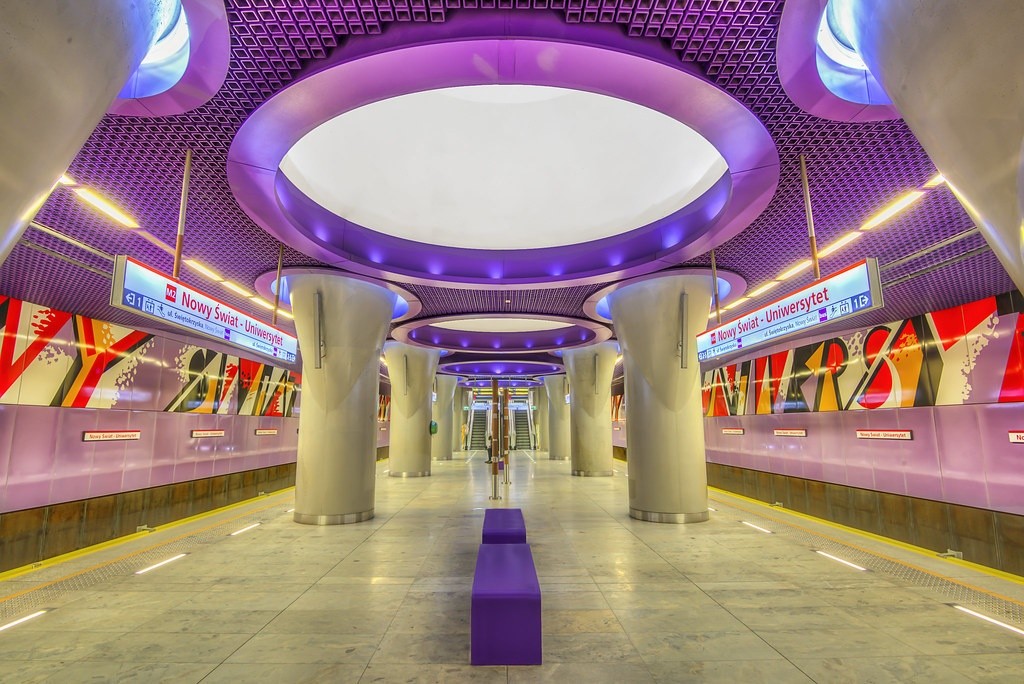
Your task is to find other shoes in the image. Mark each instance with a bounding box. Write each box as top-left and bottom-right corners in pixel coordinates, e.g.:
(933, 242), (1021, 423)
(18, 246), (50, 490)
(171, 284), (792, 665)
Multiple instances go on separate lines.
(485, 460), (492, 464)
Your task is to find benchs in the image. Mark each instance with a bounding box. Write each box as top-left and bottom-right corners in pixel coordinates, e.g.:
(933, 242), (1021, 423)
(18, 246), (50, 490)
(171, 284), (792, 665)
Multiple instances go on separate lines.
(481, 508), (527, 544)
(470, 544), (542, 666)
(499, 460), (504, 470)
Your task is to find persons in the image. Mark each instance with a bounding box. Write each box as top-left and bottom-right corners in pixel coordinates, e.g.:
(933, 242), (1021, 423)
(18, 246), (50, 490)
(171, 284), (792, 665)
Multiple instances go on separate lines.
(484, 435), (492, 464)
